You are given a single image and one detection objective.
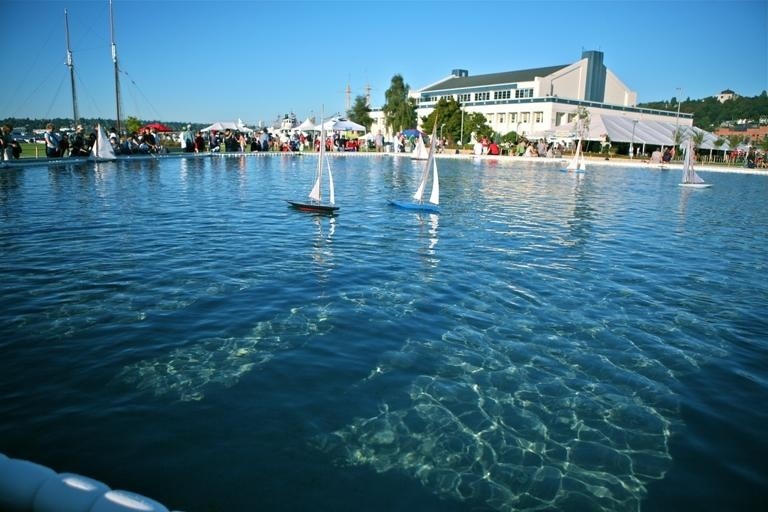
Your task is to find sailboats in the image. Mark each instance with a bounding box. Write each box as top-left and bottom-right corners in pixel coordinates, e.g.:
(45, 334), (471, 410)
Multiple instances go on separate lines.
(677, 133), (714, 189)
(558, 129), (593, 174)
(287, 104), (340, 212)
(88, 122), (117, 162)
(387, 110), (445, 214)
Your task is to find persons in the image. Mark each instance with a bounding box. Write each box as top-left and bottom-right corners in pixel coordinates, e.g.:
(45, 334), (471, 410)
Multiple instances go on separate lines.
(474, 135), (567, 157)
(44, 122), (162, 159)
(730, 147), (768, 168)
(0, 123), (18, 161)
(634, 143), (673, 164)
(178, 124), (449, 155)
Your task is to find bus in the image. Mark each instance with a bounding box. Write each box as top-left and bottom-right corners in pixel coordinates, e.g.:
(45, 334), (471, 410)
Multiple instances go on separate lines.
(10, 125), (36, 143)
(32, 127), (76, 144)
